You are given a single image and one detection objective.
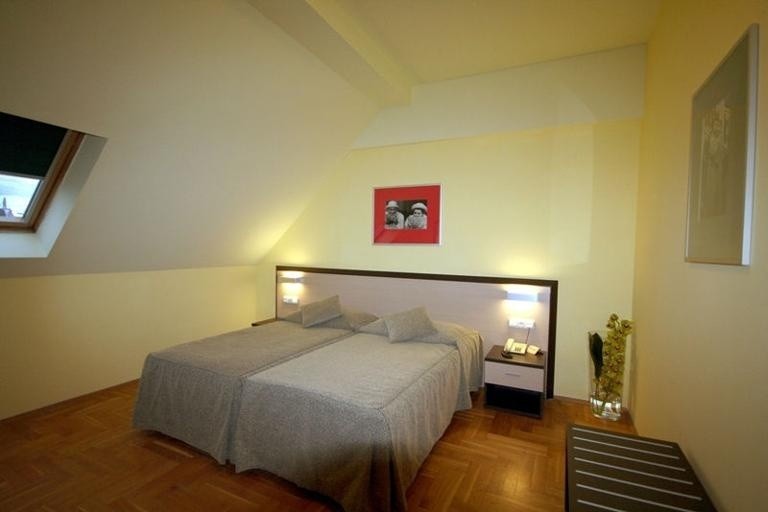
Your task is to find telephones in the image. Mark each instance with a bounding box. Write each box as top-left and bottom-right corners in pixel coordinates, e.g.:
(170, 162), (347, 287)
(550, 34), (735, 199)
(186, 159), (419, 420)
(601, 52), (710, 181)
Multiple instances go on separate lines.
(504, 338), (528, 355)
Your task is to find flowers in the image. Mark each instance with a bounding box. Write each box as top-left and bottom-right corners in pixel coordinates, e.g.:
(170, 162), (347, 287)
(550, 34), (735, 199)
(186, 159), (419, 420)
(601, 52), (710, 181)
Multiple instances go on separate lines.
(589, 314), (633, 415)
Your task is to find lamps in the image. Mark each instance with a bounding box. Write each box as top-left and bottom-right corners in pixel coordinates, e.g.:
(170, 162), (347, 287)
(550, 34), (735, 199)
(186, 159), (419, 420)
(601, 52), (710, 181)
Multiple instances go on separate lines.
(509, 320), (535, 344)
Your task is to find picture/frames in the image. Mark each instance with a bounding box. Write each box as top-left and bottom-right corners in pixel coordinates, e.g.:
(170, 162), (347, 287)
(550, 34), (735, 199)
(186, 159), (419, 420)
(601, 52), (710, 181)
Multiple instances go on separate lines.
(372, 184), (442, 246)
(684, 22), (759, 266)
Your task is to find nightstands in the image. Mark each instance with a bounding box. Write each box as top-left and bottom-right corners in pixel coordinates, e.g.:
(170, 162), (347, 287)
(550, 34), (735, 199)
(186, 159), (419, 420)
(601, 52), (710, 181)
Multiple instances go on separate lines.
(251, 318), (275, 326)
(484, 345), (546, 420)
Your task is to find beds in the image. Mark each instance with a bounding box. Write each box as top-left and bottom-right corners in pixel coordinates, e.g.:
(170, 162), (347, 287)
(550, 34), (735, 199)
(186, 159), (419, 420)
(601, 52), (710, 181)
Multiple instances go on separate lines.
(132, 266), (558, 512)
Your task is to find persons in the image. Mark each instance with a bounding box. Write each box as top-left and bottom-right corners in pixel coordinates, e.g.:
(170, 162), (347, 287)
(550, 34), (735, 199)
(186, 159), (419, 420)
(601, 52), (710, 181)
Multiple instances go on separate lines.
(385, 201), (404, 229)
(404, 202), (428, 230)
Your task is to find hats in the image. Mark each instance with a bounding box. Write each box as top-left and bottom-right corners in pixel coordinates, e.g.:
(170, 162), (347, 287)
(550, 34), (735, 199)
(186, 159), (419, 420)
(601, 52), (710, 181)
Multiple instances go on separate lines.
(411, 203), (428, 213)
(385, 201), (399, 211)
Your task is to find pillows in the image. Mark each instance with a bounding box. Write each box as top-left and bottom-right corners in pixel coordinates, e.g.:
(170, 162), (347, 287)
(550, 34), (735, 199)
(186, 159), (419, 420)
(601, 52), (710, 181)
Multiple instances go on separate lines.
(284, 294), (471, 345)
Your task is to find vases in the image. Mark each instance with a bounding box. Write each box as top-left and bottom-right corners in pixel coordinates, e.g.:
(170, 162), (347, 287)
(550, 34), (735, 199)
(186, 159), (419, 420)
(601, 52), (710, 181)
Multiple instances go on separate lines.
(590, 391), (622, 421)
